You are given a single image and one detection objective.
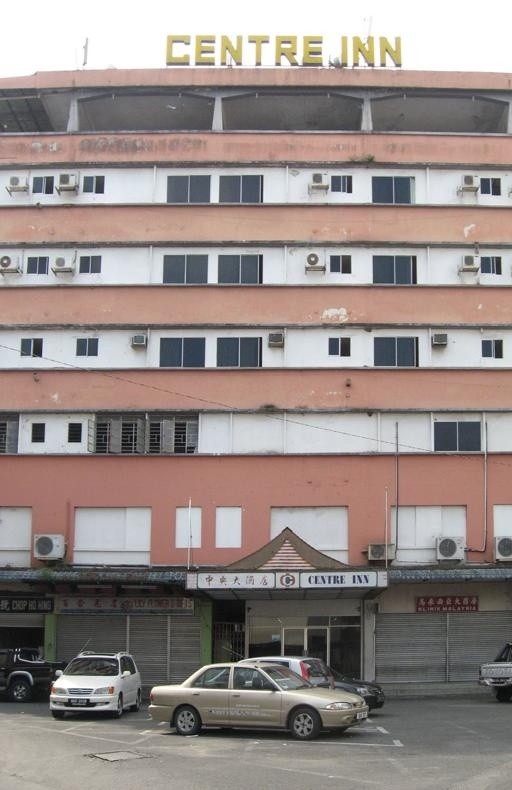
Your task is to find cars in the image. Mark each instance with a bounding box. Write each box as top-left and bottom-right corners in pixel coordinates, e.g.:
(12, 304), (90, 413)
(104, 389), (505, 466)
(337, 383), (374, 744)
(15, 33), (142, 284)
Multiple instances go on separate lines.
(141, 657), (373, 742)
(323, 661), (386, 715)
(48, 636), (142, 720)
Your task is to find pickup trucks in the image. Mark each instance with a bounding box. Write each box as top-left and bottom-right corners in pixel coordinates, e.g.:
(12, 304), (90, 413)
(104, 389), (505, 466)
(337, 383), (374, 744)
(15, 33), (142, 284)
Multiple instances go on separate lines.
(478, 643), (512, 703)
(0, 647), (68, 704)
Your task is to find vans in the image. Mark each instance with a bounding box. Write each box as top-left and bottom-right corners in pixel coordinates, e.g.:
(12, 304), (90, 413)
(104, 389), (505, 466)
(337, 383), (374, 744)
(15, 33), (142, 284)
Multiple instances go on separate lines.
(206, 654), (336, 691)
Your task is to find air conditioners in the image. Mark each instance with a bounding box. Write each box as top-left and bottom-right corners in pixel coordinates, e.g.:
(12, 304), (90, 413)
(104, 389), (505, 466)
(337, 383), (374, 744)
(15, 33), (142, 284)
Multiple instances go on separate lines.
(30, 533), (68, 565)
(436, 535), (466, 563)
(366, 539), (398, 563)
(492, 535), (511, 565)
(8, 174), (27, 192)
(0, 253), (20, 275)
(132, 333), (147, 349)
(303, 250), (329, 272)
(307, 171), (330, 191)
(457, 172), (481, 194)
(50, 254), (74, 273)
(56, 171), (78, 191)
(429, 333), (449, 349)
(267, 332), (284, 349)
(457, 254), (480, 273)
